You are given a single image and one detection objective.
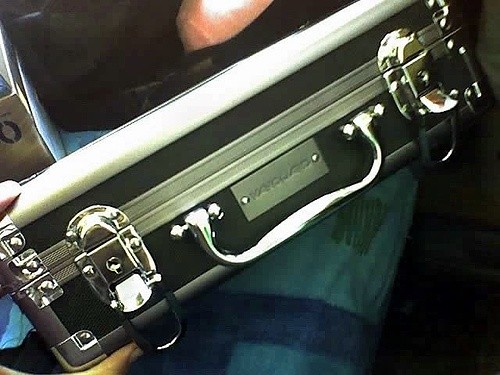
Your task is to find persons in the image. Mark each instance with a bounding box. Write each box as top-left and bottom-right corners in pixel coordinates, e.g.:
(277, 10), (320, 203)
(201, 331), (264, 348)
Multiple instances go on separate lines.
(0, 178), (22, 217)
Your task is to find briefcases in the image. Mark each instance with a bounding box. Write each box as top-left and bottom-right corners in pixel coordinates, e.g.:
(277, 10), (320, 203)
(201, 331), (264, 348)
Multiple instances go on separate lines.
(2, 1), (491, 373)
